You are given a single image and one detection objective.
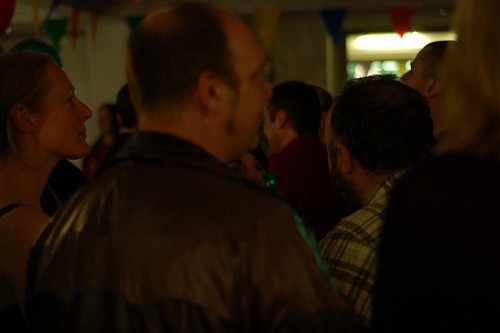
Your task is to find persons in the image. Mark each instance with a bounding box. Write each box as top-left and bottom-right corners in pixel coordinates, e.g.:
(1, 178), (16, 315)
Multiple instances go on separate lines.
(0, 49), (94, 332)
(399, 40), (467, 153)
(8, 38), (356, 235)
(31, 3), (357, 333)
(372, 0), (499, 333)
(317, 74), (447, 333)
